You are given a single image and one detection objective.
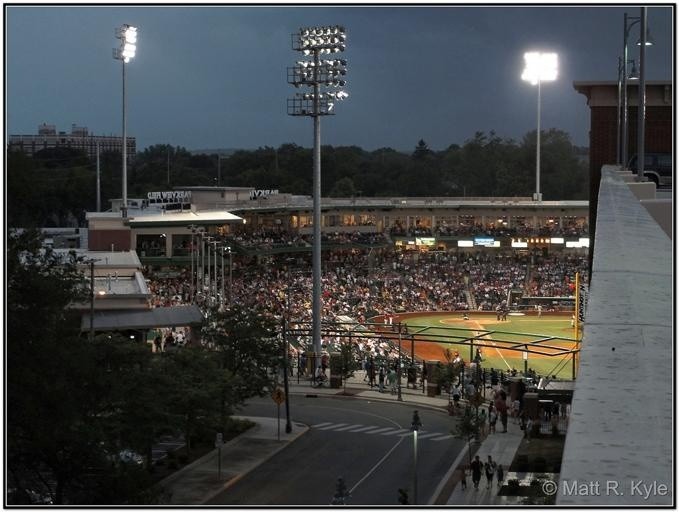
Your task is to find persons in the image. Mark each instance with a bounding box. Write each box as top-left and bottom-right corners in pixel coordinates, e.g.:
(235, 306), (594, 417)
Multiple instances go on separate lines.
(332, 477), (353, 505)
(484, 455), (496, 489)
(398, 489), (410, 504)
(497, 463), (504, 486)
(460, 469), (467, 491)
(141, 212), (588, 433)
(470, 455), (483, 489)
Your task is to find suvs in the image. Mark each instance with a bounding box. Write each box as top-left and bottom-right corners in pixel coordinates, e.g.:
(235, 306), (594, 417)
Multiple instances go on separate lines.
(627, 152), (671, 189)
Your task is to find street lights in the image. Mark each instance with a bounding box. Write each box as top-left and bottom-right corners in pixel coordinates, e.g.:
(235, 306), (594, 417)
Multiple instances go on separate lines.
(410, 410), (422, 505)
(188, 224), (236, 314)
(287, 23), (347, 369)
(621, 13), (654, 167)
(110, 23), (138, 216)
(520, 48), (561, 199)
